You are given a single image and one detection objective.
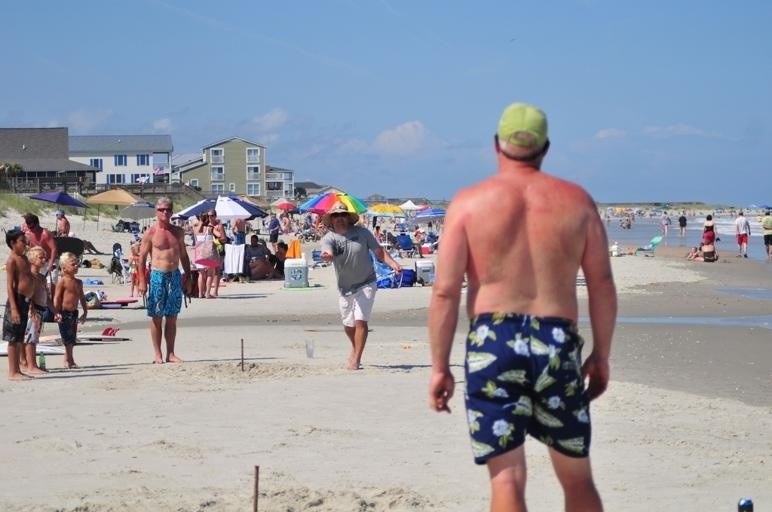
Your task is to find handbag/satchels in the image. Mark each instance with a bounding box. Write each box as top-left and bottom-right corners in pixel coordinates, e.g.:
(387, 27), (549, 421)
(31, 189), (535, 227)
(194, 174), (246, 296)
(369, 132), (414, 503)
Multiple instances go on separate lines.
(42, 306), (54, 322)
(195, 235), (221, 268)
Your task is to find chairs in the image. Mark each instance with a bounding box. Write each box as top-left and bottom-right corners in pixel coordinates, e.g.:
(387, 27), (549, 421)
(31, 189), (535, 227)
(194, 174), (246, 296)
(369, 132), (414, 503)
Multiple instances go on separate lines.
(370, 251), (404, 289)
(633, 236), (663, 257)
(397, 235), (417, 259)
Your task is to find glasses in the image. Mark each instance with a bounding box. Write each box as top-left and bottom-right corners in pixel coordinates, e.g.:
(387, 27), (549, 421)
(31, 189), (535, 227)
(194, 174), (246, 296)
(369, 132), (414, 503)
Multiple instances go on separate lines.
(157, 208), (172, 213)
(330, 213), (349, 217)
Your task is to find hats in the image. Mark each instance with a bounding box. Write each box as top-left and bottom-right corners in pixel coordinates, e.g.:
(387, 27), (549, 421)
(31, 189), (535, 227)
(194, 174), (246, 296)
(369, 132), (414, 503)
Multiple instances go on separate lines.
(322, 202), (360, 225)
(56, 210), (64, 214)
(497, 104), (548, 146)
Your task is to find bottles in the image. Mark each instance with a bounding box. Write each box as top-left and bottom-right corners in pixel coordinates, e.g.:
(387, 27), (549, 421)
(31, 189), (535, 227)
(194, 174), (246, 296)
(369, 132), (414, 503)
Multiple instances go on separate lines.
(39, 351), (46, 370)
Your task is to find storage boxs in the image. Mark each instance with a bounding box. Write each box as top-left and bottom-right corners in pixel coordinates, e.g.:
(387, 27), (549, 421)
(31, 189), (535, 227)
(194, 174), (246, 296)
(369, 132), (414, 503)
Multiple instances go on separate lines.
(109, 257), (129, 284)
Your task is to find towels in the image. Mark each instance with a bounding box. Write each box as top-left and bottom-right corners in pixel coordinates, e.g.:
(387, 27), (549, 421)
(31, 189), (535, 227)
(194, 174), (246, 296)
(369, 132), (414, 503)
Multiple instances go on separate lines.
(224, 243), (246, 274)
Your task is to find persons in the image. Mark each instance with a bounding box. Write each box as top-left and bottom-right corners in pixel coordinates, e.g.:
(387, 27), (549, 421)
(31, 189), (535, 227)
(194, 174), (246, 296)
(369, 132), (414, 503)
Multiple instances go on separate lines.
(53, 251), (88, 368)
(318, 199), (402, 374)
(1, 229), (33, 379)
(23, 244), (62, 374)
(359, 213), (441, 287)
(598, 197), (771, 262)
(137, 197), (193, 365)
(54, 210), (330, 299)
(425, 97), (620, 512)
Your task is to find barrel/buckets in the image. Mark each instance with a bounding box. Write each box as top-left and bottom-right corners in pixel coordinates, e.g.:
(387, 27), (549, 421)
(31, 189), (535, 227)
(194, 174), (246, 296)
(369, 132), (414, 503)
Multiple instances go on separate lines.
(611, 241), (621, 256)
